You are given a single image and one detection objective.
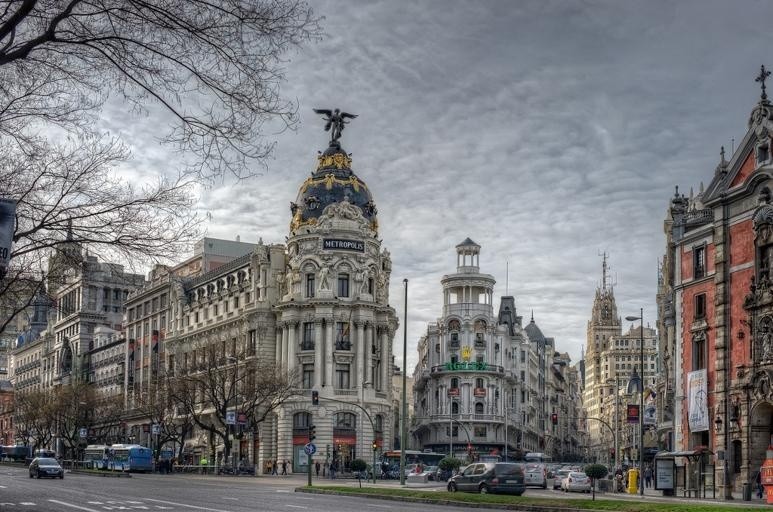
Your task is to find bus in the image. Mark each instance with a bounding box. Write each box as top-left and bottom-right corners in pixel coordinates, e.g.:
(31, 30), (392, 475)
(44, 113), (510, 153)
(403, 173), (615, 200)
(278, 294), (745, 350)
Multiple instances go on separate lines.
(381, 449), (446, 473)
(84, 444), (153, 472)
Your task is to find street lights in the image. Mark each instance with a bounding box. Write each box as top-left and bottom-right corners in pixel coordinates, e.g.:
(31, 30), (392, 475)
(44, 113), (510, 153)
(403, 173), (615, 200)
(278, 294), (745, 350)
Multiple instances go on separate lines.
(607, 375), (619, 493)
(227, 358), (238, 440)
(77, 402), (87, 467)
(625, 308), (643, 496)
(360, 381), (374, 461)
(130, 435), (136, 444)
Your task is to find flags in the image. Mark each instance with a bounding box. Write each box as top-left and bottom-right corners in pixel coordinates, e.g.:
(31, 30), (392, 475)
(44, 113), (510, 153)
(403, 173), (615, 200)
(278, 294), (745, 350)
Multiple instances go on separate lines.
(685, 369), (709, 433)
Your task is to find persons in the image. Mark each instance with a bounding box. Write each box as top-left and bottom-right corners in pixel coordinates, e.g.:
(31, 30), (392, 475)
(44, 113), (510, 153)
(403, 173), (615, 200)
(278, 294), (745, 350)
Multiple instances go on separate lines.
(315, 460), (320, 476)
(614, 464), (654, 488)
(755, 471), (764, 499)
(160, 456), (186, 473)
(200, 456), (207, 474)
(321, 108), (350, 143)
(329, 460), (336, 480)
(266, 459), (272, 472)
(280, 458), (286, 475)
(271, 460), (278, 475)
(285, 460), (292, 474)
(414, 463), (422, 473)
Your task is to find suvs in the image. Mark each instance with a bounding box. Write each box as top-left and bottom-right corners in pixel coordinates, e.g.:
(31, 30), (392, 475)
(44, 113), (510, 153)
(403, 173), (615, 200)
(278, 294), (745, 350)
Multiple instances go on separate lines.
(221, 457), (252, 475)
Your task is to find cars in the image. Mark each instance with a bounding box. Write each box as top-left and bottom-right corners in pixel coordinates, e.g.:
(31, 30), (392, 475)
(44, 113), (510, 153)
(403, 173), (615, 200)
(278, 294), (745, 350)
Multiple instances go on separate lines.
(352, 463), (466, 479)
(447, 464), (526, 497)
(28, 449), (63, 478)
(521, 452), (593, 493)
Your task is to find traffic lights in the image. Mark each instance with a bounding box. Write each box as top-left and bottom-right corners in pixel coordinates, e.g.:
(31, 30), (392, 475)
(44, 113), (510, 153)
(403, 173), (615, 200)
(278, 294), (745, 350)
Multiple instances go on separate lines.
(553, 414), (557, 426)
(309, 426), (315, 440)
(312, 391), (319, 405)
(468, 447), (471, 455)
(372, 440), (376, 450)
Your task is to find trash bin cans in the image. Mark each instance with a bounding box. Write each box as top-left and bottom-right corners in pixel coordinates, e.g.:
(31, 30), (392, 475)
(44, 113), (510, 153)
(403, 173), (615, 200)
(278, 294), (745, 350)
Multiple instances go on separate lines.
(160, 463), (167, 474)
(743, 484), (751, 501)
